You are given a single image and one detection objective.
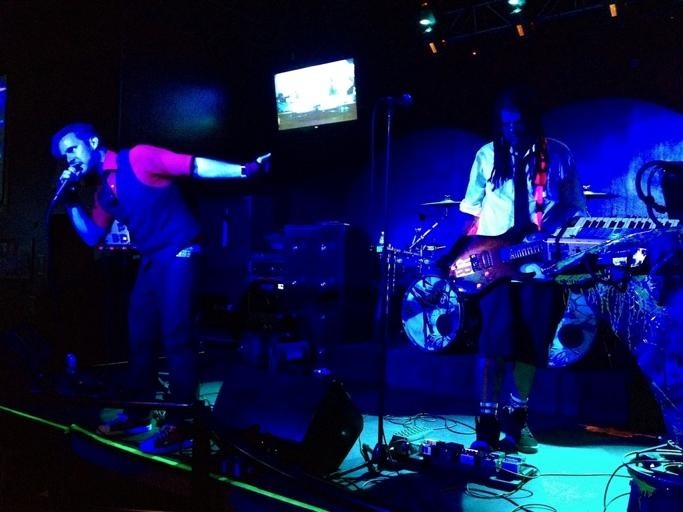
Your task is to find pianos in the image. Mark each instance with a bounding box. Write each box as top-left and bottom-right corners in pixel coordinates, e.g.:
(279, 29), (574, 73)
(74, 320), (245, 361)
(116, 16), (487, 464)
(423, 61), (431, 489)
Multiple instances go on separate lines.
(549, 215), (680, 240)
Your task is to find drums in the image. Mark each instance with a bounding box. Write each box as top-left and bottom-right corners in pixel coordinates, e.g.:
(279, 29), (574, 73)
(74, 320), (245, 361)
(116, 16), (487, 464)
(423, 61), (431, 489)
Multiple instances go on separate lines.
(402, 277), (466, 352)
(547, 288), (599, 368)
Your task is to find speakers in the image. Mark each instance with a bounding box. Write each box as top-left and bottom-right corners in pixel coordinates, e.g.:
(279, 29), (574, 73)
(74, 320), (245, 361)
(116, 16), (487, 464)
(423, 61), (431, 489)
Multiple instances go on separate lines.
(210, 361), (362, 475)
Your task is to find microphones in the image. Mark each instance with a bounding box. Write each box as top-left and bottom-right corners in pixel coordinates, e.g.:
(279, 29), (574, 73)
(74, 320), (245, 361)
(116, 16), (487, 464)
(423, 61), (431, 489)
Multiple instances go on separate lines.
(583, 189), (615, 204)
(52, 165), (79, 203)
(380, 92), (413, 107)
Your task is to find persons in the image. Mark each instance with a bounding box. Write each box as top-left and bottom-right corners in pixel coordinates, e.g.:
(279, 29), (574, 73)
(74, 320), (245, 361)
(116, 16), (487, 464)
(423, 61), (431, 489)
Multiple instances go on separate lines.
(458, 90), (587, 451)
(49, 123), (272, 458)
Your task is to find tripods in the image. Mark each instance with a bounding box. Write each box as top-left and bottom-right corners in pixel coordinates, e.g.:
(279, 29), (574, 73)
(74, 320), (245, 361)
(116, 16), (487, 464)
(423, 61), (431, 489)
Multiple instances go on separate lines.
(340, 145), (443, 480)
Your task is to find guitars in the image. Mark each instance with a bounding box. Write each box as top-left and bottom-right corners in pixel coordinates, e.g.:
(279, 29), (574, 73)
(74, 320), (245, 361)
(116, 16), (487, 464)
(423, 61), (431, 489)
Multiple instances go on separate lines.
(445, 223), (545, 300)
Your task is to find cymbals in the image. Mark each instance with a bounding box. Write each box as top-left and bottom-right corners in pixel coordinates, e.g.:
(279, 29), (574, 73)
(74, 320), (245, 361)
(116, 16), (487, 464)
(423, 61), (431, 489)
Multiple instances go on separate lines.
(422, 196), (461, 208)
(584, 185), (607, 197)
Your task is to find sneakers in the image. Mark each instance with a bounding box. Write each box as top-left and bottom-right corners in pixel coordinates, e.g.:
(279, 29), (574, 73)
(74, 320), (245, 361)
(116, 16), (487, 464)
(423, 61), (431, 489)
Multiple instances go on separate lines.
(141, 426), (193, 455)
(97, 413), (152, 437)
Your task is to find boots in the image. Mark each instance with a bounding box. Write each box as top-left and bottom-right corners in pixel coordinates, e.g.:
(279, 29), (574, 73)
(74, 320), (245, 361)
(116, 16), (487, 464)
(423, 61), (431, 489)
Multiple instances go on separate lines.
(475, 412), (499, 452)
(505, 406), (540, 454)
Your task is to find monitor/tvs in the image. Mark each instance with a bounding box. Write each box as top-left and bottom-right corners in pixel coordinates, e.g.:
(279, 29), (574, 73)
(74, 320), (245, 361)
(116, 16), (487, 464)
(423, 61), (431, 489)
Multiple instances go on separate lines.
(270, 57), (363, 134)
